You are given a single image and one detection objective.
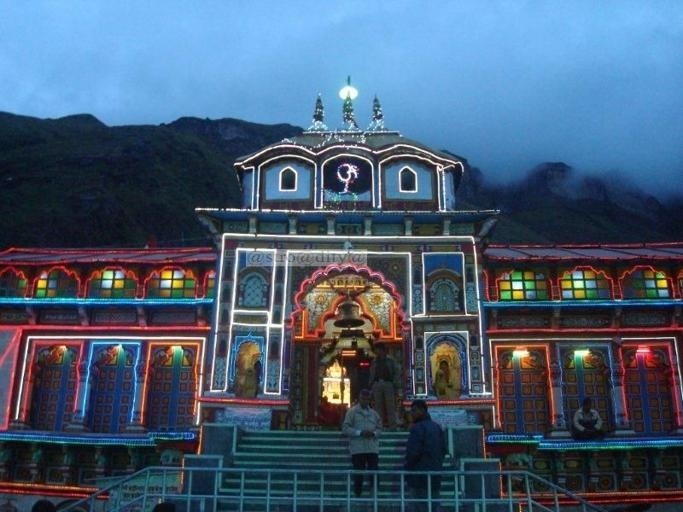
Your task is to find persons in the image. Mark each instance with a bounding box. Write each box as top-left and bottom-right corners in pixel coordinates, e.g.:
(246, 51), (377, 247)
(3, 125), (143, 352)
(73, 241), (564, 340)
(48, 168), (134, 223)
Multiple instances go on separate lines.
(153, 503), (175, 512)
(432, 360), (453, 399)
(571, 396), (606, 441)
(342, 388), (383, 498)
(31, 500), (57, 511)
(404, 401), (445, 511)
(369, 343), (402, 433)
